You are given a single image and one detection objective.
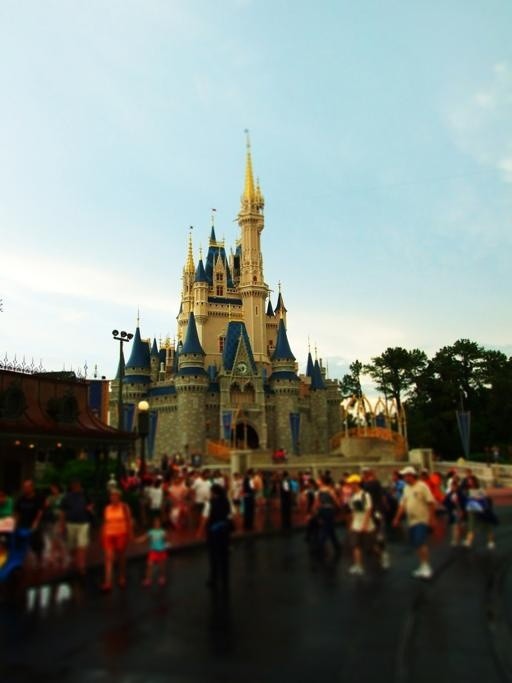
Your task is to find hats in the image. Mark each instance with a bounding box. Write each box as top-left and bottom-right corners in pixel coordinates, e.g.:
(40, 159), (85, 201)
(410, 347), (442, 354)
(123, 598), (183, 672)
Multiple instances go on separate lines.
(346, 474), (361, 483)
(399, 468), (415, 475)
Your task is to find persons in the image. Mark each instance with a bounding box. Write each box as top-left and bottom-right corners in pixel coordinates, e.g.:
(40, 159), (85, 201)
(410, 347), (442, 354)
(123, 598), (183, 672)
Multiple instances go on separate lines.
(1, 447), (499, 589)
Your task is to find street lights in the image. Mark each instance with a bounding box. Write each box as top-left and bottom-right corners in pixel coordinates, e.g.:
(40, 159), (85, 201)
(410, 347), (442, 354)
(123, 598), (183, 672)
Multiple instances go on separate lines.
(112, 329), (150, 464)
